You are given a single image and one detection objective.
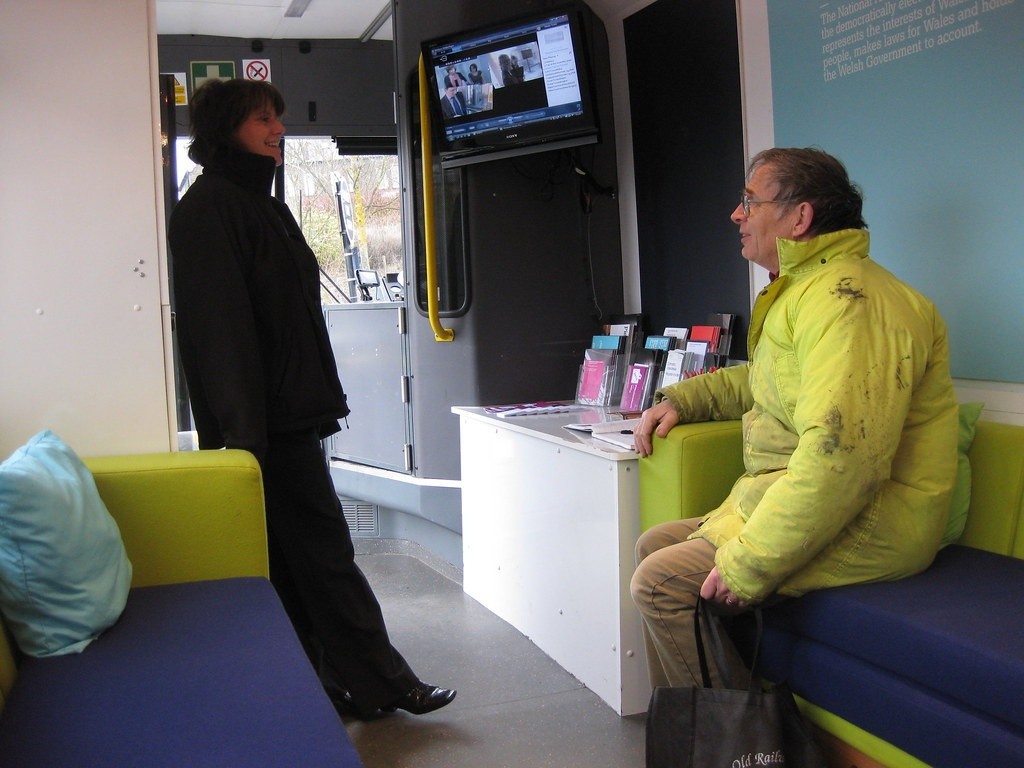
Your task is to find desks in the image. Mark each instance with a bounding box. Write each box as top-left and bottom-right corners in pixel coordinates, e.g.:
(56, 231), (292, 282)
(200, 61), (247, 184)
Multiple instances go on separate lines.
(451, 402), (653, 717)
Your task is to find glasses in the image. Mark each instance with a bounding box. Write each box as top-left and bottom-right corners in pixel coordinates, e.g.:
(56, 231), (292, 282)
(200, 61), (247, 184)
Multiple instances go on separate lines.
(739, 189), (802, 218)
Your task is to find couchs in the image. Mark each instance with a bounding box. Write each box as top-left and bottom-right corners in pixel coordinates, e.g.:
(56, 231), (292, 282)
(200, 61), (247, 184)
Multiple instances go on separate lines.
(638, 420), (1024, 768)
(0, 450), (363, 767)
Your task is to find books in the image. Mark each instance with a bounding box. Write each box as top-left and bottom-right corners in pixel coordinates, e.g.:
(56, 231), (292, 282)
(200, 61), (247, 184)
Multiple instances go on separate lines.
(565, 418), (642, 450)
(578, 313), (734, 410)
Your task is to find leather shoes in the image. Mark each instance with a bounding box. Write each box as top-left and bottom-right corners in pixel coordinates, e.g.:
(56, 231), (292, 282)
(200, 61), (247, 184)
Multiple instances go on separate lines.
(379, 680), (457, 715)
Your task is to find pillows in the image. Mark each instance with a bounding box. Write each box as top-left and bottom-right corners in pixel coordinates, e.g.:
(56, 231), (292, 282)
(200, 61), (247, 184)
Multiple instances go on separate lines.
(0, 429), (133, 658)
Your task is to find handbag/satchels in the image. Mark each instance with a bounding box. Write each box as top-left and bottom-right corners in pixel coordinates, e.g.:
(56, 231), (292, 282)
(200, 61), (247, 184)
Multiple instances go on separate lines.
(644, 590), (828, 768)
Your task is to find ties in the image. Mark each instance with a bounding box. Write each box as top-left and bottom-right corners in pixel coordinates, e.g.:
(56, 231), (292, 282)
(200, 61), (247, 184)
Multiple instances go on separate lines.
(451, 96), (463, 116)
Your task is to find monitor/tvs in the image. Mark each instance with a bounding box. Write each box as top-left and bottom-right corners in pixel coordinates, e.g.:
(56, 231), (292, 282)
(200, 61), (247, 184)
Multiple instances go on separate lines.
(421, 0), (602, 171)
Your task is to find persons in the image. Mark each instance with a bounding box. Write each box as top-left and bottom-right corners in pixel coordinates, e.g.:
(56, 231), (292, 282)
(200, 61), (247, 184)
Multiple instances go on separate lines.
(172, 79), (456, 720)
(440, 63), (483, 118)
(498, 54), (525, 86)
(631, 147), (960, 692)
